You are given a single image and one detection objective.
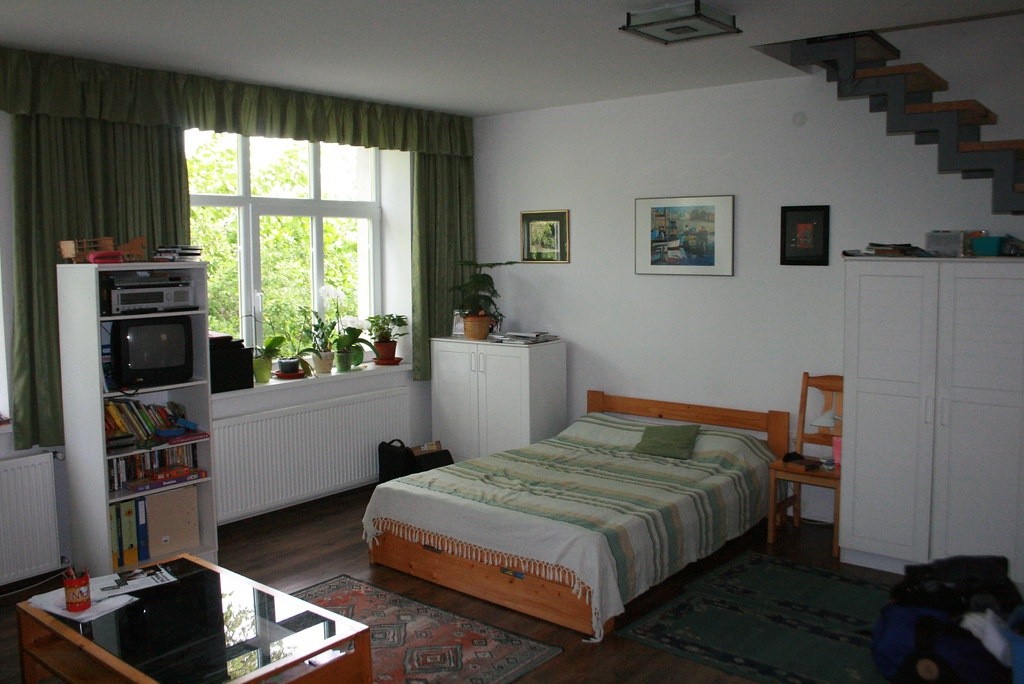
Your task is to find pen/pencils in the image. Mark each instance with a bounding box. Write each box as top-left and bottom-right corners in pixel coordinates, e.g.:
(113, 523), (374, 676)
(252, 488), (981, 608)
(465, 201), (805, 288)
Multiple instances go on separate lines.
(64, 567), (76, 579)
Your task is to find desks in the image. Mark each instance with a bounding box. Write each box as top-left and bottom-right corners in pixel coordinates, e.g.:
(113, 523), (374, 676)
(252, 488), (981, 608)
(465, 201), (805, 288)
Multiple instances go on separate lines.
(16, 552), (374, 684)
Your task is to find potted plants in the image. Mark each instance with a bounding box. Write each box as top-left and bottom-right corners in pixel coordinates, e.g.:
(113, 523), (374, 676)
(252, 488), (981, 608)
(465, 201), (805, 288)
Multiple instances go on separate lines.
(447, 260), (521, 341)
(265, 314), (323, 379)
(300, 306), (340, 373)
(330, 325), (382, 372)
(249, 334), (287, 382)
(364, 313), (410, 366)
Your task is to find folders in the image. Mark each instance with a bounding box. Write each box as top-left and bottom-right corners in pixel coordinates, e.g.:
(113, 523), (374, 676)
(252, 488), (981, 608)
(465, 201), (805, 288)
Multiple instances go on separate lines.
(135, 497), (148, 560)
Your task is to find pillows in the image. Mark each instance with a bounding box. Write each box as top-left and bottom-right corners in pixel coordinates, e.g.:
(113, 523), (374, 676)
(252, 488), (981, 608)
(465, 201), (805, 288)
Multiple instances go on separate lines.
(631, 423), (702, 461)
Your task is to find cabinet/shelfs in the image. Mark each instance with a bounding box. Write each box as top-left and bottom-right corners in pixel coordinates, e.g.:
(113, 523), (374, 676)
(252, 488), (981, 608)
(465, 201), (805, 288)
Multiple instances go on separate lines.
(838, 255), (1023, 590)
(55, 260), (219, 577)
(426, 338), (568, 464)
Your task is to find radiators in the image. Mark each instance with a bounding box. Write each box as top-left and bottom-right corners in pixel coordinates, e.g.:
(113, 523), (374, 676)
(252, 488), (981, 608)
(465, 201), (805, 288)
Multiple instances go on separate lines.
(213, 384), (410, 526)
(0, 451), (65, 585)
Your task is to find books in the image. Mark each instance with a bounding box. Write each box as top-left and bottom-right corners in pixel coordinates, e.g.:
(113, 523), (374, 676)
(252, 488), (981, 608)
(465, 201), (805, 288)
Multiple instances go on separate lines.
(154, 244), (202, 262)
(104, 398), (208, 493)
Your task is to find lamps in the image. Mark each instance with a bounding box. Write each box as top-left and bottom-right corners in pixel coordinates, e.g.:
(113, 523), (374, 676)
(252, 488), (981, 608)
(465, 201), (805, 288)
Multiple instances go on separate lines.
(619, 1), (743, 45)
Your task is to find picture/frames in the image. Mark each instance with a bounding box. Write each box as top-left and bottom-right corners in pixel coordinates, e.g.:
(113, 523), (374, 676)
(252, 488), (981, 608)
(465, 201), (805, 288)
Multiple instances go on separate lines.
(518, 210), (569, 264)
(633, 195), (735, 277)
(780, 205), (830, 266)
(451, 309), (471, 338)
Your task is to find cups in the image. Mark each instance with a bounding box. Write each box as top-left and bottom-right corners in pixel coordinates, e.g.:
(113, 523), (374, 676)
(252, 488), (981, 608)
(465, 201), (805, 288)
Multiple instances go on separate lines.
(63, 573), (91, 612)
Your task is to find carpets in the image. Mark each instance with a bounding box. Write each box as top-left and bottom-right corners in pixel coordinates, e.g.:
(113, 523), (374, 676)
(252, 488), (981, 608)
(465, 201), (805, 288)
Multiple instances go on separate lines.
(287, 573), (564, 684)
(608, 548), (895, 684)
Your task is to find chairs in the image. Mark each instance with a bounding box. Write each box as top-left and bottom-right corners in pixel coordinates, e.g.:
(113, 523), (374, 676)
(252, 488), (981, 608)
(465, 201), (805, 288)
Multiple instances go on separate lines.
(766, 372), (845, 558)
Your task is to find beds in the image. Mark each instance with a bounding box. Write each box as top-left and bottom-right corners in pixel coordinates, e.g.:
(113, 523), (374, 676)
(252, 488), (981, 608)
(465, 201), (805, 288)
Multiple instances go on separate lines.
(363, 389), (790, 644)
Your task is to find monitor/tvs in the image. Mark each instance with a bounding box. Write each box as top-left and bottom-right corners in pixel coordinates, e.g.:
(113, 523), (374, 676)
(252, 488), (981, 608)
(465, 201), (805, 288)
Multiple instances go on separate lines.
(110, 315), (193, 391)
